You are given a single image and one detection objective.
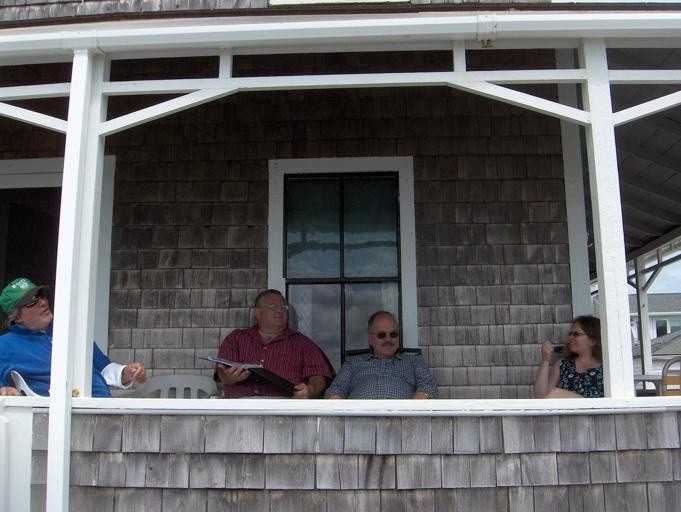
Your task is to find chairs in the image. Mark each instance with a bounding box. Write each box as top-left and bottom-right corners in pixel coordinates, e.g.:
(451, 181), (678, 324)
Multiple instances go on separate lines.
(9, 369), (51, 396)
(136, 372), (218, 399)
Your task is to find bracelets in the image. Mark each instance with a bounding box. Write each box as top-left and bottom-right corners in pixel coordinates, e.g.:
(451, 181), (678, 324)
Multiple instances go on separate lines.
(540, 362), (548, 367)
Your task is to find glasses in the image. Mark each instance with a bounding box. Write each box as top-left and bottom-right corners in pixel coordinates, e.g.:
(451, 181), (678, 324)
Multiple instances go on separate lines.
(568, 332), (588, 337)
(268, 303), (289, 312)
(24, 292), (47, 307)
(376, 331), (398, 340)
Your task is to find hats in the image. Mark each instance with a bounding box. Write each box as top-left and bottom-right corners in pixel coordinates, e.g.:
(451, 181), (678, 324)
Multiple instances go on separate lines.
(0, 277), (50, 315)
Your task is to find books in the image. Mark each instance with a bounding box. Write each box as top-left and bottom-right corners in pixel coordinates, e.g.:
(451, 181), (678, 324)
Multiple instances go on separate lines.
(197, 355), (294, 389)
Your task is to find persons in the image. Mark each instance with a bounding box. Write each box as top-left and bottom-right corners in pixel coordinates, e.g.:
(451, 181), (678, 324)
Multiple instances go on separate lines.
(212, 288), (335, 400)
(533, 314), (605, 399)
(324, 310), (439, 400)
(0, 276), (147, 399)
